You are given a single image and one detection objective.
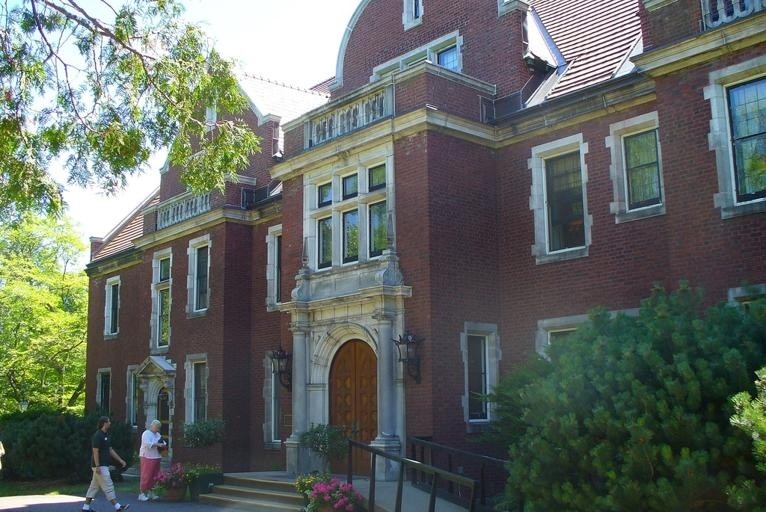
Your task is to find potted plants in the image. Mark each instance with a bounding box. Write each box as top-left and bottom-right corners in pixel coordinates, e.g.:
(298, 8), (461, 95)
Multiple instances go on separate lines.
(179, 418), (232, 470)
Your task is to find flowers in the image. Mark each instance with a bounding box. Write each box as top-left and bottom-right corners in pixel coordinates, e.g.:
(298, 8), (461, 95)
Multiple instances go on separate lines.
(150, 461), (223, 504)
(295, 470), (364, 512)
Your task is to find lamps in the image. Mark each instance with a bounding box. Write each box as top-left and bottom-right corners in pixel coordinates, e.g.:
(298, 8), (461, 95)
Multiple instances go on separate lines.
(266, 344), (293, 392)
(388, 327), (426, 386)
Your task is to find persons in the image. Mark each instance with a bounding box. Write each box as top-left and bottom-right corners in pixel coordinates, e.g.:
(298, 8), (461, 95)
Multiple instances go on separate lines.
(0, 441), (6, 470)
(138, 420), (167, 501)
(81, 416), (131, 512)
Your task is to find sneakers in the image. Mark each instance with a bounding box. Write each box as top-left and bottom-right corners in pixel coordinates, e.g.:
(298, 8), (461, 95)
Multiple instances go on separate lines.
(147, 492), (160, 500)
(115, 502), (130, 512)
(81, 508), (96, 512)
(137, 494), (149, 501)
